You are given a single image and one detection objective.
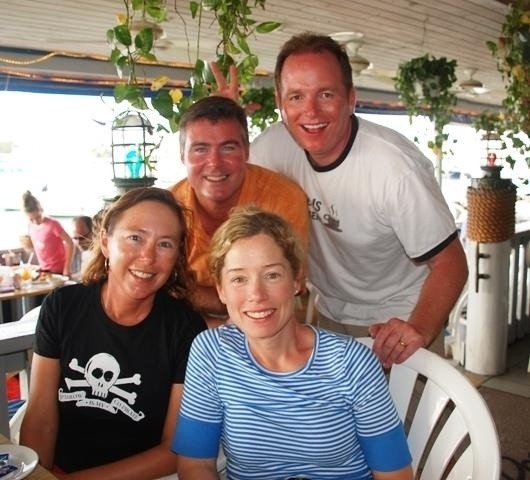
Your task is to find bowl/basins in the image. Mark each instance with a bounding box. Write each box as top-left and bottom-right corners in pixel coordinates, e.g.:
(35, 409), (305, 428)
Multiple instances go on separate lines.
(50, 278), (65, 288)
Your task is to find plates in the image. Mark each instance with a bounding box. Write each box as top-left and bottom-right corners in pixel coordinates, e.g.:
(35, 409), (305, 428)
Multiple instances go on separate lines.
(0, 444), (39, 480)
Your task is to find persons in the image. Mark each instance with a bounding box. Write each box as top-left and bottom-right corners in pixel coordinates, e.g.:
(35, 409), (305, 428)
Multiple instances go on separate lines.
(72, 216), (92, 272)
(171, 205), (414, 480)
(166, 96), (309, 329)
(19, 190), (74, 279)
(209, 32), (469, 369)
(19, 187), (208, 480)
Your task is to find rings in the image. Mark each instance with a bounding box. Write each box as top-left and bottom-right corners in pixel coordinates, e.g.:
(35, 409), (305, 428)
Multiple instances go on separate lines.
(399, 340), (407, 348)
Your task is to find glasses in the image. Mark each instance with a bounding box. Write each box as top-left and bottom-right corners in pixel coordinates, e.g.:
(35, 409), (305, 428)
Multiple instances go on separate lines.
(73, 231), (90, 240)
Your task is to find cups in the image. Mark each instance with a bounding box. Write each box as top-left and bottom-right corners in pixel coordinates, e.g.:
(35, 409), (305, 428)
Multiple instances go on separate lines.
(18, 264), (32, 289)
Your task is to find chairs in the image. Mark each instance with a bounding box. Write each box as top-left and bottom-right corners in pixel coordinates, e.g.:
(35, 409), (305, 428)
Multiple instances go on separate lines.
(354, 334), (501, 480)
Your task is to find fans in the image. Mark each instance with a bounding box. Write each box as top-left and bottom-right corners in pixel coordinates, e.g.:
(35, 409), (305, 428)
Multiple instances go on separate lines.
(449, 68), (499, 97)
(345, 36), (398, 86)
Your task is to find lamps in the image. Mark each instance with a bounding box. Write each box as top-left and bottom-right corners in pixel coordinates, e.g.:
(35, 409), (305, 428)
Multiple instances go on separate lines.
(479, 132), (506, 178)
(111, 107), (158, 193)
